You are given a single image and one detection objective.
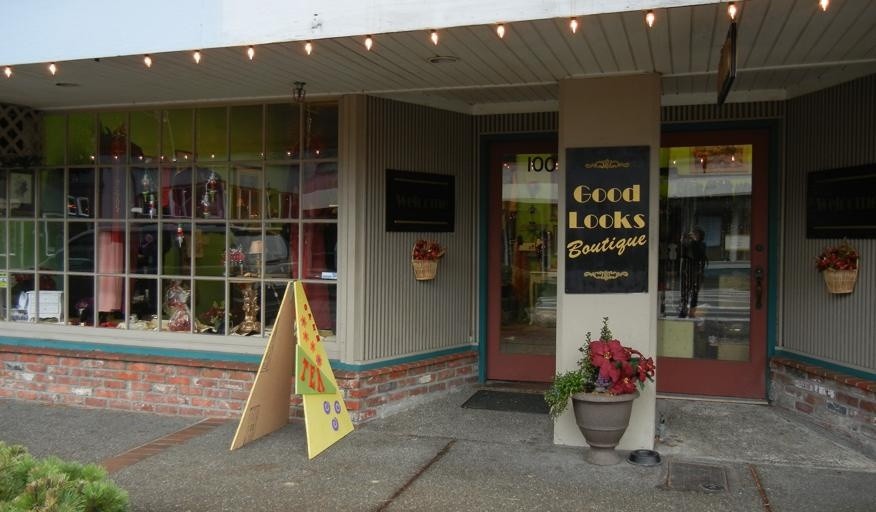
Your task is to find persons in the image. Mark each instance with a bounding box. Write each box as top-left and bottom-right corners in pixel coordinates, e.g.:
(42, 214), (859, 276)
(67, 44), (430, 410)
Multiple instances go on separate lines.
(676, 226), (708, 320)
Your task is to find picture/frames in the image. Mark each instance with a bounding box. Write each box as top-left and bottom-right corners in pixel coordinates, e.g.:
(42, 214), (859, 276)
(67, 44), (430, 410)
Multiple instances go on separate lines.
(231, 164), (263, 237)
(687, 144), (750, 174)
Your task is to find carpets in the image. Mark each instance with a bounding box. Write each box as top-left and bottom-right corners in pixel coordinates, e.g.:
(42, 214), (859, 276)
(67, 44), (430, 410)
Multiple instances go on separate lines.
(458, 388), (550, 415)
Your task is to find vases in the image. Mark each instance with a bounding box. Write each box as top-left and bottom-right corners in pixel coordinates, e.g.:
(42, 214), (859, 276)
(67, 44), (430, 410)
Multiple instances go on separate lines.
(570, 384), (639, 466)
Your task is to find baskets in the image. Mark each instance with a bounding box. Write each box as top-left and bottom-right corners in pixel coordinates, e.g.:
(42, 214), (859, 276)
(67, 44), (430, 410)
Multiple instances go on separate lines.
(411, 241), (439, 281)
(823, 249), (859, 294)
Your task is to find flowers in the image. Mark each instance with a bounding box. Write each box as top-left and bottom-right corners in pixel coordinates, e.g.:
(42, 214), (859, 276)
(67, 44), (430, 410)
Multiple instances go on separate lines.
(409, 239), (444, 260)
(812, 245), (858, 275)
(540, 318), (659, 418)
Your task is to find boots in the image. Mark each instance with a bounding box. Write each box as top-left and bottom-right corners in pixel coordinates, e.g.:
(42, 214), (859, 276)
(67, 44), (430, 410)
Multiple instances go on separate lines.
(679, 304), (696, 318)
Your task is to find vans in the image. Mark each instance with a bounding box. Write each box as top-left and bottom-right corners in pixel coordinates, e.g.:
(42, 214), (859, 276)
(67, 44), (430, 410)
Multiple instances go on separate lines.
(661, 257), (754, 338)
(5, 223), (293, 332)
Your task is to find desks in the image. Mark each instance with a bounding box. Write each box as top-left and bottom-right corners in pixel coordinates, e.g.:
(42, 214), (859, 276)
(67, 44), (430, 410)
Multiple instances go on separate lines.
(527, 269), (558, 326)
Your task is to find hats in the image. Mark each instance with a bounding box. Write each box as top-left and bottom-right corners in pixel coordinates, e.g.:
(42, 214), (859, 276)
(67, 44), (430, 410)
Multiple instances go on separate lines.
(689, 227), (705, 241)
